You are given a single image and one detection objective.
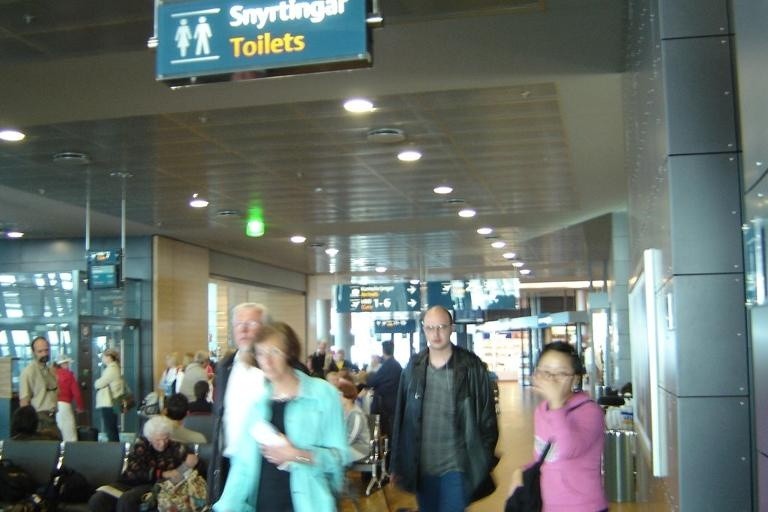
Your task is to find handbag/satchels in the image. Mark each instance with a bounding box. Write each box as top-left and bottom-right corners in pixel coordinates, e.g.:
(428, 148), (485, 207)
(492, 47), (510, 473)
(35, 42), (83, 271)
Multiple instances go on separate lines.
(157, 468), (210, 512)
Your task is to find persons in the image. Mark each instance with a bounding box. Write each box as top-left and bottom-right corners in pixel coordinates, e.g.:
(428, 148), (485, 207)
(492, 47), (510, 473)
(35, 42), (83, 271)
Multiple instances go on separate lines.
(211, 323), (348, 512)
(92, 351), (125, 442)
(306, 338), (338, 378)
(213, 304), (270, 505)
(510, 342), (606, 512)
(360, 340), (403, 477)
(53, 356), (83, 444)
(329, 349), (359, 376)
(159, 352), (179, 413)
(389, 306), (502, 512)
(195, 350), (215, 402)
(176, 354), (213, 404)
(338, 380), (371, 468)
(3, 405), (61, 440)
(165, 393), (206, 445)
(187, 381), (215, 414)
(91, 416), (205, 512)
(18, 336), (63, 442)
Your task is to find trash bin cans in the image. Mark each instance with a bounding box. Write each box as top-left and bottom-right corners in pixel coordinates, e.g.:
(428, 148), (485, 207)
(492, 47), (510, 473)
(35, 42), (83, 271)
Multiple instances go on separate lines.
(603, 427), (639, 505)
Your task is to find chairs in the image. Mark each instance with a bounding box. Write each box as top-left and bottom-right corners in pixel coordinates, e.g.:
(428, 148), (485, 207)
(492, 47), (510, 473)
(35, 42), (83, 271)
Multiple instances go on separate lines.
(182, 415), (220, 489)
(190, 441), (223, 512)
(49, 441), (128, 509)
(1, 437), (64, 487)
(348, 414), (390, 495)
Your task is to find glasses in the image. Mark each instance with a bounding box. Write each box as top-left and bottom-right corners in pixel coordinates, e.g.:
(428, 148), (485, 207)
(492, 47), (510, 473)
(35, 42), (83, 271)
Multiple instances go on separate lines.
(423, 323), (451, 330)
(252, 347), (287, 359)
(536, 370), (576, 379)
(231, 320), (262, 328)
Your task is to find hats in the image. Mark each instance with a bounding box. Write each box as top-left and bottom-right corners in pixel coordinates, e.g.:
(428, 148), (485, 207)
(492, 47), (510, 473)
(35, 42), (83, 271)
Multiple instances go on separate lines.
(54, 354), (73, 365)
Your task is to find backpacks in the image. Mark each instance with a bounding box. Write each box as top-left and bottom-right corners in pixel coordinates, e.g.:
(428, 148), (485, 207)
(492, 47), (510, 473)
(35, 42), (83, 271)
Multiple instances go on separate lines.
(0, 457), (37, 511)
(41, 465), (90, 512)
